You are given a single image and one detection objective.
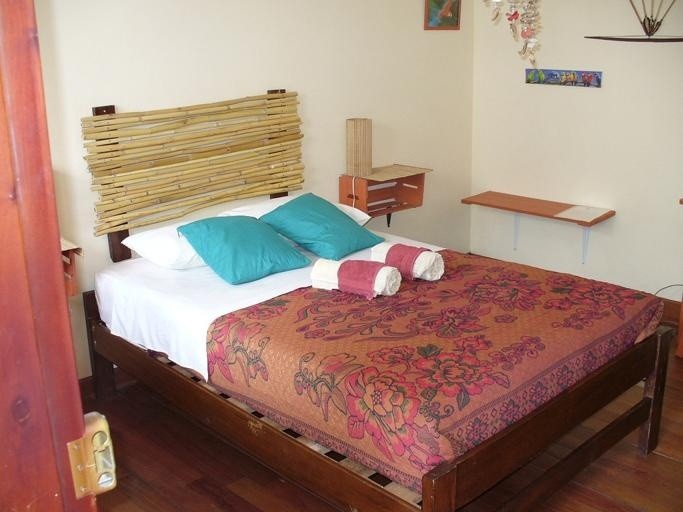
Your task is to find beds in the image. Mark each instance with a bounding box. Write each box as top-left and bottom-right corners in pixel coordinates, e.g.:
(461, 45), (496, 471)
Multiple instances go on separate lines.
(80, 89), (673, 511)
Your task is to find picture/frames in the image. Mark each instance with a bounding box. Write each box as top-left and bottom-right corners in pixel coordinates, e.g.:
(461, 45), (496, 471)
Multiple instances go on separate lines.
(424, 0), (461, 30)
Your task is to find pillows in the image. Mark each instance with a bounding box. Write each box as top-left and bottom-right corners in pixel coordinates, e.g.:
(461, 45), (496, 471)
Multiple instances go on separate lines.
(260, 201), (373, 247)
(258, 192), (385, 261)
(176, 215), (312, 285)
(121, 220), (296, 269)
